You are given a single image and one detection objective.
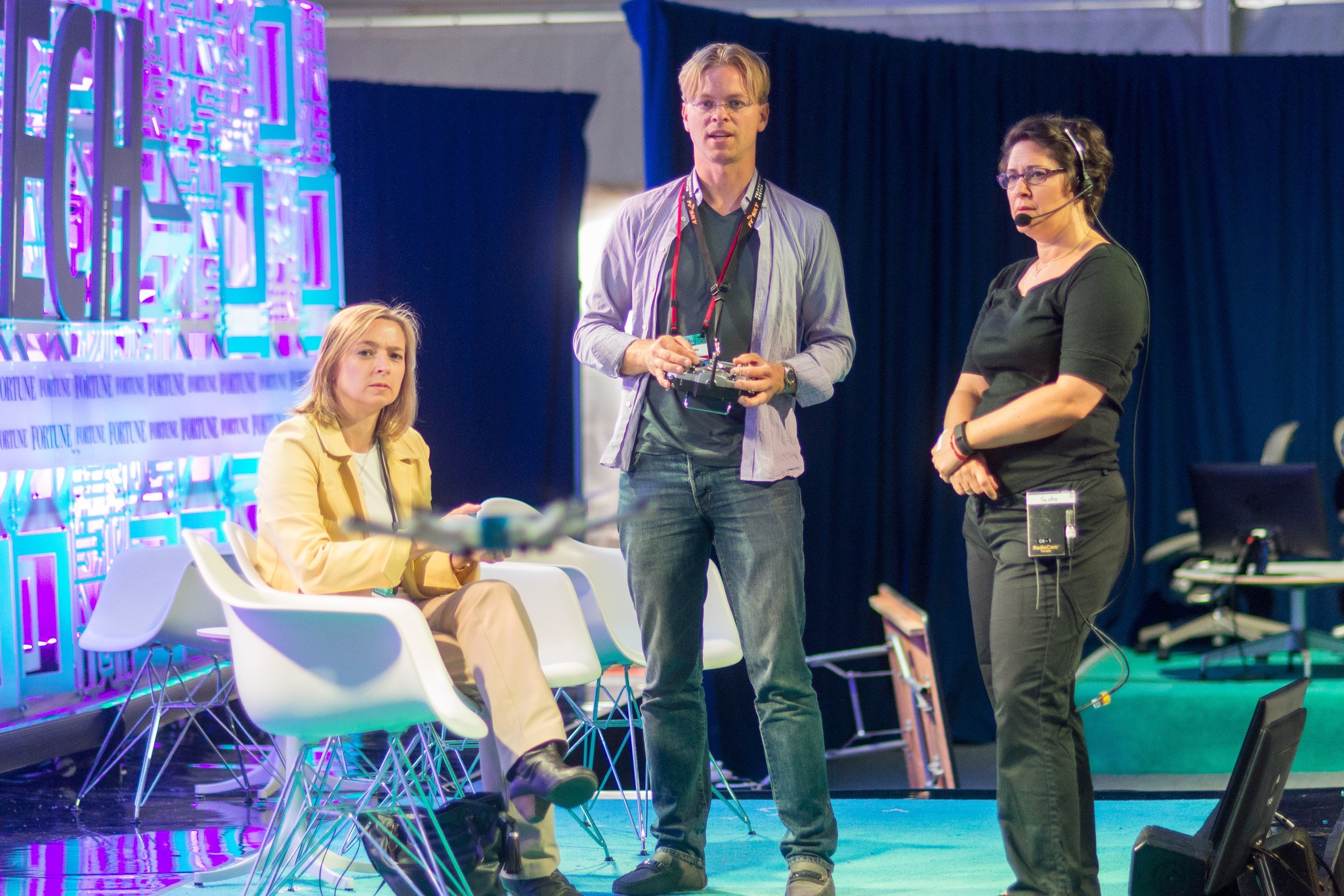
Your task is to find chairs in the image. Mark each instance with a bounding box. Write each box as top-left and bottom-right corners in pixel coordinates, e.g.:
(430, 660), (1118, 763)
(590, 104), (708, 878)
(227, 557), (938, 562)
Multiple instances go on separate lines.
(71, 496), (758, 896)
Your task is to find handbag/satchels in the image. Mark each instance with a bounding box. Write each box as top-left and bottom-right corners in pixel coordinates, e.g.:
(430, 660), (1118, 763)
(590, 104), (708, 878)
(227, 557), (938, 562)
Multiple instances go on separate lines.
(356, 791), (523, 896)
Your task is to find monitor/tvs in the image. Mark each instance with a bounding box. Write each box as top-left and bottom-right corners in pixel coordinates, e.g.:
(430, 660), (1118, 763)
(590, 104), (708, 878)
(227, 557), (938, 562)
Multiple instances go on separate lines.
(1193, 465), (1331, 574)
(1205, 676), (1320, 894)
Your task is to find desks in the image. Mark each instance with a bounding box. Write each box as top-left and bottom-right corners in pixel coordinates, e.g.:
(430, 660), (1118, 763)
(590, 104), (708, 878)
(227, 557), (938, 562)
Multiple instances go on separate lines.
(1174, 562), (1344, 656)
(805, 580), (959, 791)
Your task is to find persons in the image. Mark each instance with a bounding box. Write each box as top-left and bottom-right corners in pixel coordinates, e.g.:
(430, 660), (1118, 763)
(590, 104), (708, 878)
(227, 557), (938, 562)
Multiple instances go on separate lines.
(573, 42), (858, 896)
(253, 303), (597, 896)
(930, 114), (1152, 896)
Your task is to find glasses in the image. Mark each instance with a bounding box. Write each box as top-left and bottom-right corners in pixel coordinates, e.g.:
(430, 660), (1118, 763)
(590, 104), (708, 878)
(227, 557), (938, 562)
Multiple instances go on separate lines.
(686, 98), (757, 112)
(997, 167), (1067, 189)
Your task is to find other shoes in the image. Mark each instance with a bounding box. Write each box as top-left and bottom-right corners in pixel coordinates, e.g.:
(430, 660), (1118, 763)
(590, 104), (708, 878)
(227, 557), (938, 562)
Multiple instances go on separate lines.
(612, 853), (708, 896)
(785, 862), (835, 896)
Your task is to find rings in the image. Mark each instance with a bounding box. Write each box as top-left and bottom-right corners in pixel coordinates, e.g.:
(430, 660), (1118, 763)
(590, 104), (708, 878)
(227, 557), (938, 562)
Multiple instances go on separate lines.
(964, 486), (971, 491)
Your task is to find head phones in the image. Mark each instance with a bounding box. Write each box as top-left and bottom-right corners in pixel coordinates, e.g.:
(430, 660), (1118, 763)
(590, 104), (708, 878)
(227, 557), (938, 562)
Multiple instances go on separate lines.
(1062, 129), (1095, 198)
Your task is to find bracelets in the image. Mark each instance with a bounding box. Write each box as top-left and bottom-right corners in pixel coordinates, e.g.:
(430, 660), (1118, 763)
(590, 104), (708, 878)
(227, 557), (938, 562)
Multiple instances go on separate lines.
(950, 432), (968, 462)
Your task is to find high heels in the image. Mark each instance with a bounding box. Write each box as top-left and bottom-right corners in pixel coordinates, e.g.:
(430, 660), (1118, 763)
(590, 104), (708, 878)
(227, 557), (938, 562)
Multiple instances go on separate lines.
(509, 744), (598, 822)
(499, 869), (584, 896)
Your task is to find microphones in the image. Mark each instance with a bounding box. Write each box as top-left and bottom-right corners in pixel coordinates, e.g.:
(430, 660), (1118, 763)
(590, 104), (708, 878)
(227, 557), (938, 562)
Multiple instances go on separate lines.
(1015, 185), (1092, 228)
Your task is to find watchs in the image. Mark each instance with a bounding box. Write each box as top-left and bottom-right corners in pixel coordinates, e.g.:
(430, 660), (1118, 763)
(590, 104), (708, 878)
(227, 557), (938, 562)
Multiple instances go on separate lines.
(954, 421), (975, 455)
(779, 362), (796, 394)
